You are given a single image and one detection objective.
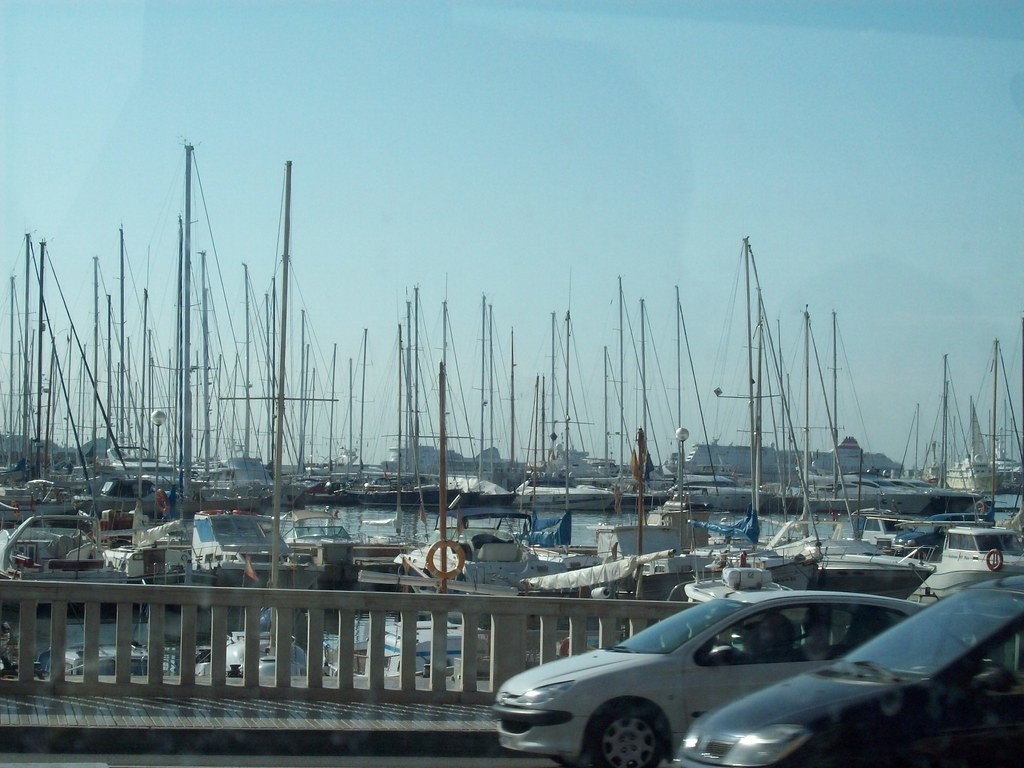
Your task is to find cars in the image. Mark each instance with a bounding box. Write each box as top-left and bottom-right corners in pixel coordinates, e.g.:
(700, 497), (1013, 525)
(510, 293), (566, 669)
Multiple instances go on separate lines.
(489, 585), (971, 767)
(671, 573), (1024, 767)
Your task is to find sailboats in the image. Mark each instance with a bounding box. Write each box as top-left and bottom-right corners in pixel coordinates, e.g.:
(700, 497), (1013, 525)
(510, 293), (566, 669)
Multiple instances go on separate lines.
(0, 139), (1024, 680)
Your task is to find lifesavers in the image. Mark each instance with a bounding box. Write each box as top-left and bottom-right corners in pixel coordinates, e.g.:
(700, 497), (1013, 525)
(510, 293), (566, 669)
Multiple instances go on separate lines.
(232, 510), (256, 516)
(426, 540), (465, 580)
(11, 500), (21, 516)
(975, 501), (986, 515)
(156, 489), (170, 513)
(199, 509), (223, 515)
(986, 548), (1003, 571)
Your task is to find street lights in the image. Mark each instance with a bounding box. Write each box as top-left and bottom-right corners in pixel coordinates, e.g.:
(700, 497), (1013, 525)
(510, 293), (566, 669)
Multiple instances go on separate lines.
(148, 409), (167, 549)
(675, 426), (689, 549)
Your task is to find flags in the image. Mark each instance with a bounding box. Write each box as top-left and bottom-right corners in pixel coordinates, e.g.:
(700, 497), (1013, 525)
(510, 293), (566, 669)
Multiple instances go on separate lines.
(244, 559), (257, 581)
(629, 448), (655, 484)
